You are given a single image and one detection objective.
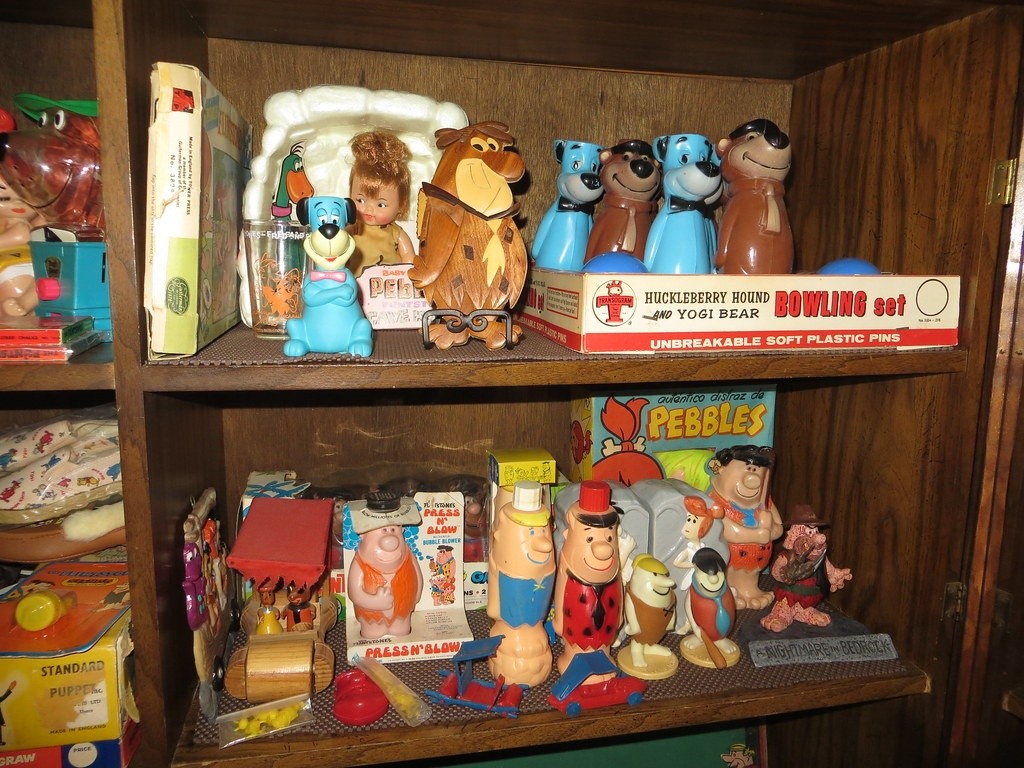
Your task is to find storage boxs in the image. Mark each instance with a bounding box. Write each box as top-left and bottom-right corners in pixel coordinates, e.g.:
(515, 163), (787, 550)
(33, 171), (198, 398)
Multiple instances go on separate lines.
(142, 61), (254, 360)
(511, 267), (962, 352)
(570, 383), (778, 495)
(462, 560), (489, 611)
(486, 448), (558, 484)
(551, 471), (572, 516)
(0, 561), (142, 768)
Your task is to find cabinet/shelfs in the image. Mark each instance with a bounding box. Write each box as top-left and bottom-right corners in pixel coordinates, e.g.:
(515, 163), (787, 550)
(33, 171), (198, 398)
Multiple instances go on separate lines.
(0, 0), (1024, 768)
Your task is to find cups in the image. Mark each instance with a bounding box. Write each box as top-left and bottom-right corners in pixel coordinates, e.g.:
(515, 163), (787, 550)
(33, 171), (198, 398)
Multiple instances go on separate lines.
(243, 219), (310, 339)
